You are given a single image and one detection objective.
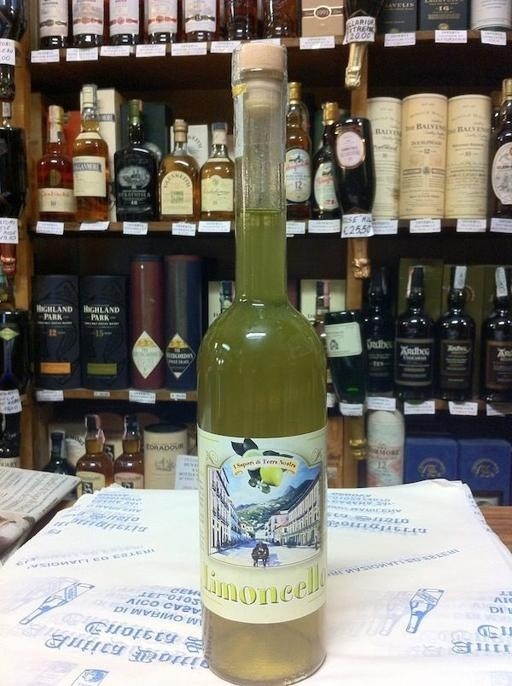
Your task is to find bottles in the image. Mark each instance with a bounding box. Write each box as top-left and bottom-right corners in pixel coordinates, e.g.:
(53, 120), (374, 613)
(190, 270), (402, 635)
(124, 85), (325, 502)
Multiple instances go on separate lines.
(41, 433), (75, 499)
(365, 97), (402, 223)
(70, 1), (105, 44)
(489, 80), (511, 219)
(407, 585), (444, 635)
(365, 409), (406, 489)
(0, 126), (29, 277)
(324, 309), (369, 401)
(481, 267), (512, 409)
(0, 411), (20, 470)
(143, 425), (188, 490)
(0, 0), (27, 119)
(361, 263), (395, 394)
(436, 266), (478, 402)
(263, 1), (301, 37)
(145, 4), (179, 40)
(310, 278), (336, 395)
(79, 272), (130, 390)
(224, 1), (257, 39)
(200, 41), (328, 686)
(447, 91), (496, 222)
(165, 252), (206, 393)
(75, 414), (115, 498)
(115, 97), (159, 218)
(399, 91), (450, 218)
(198, 119), (236, 218)
(284, 77), (314, 217)
(471, 1), (512, 28)
(113, 414), (144, 491)
(130, 253), (165, 389)
(34, 104), (75, 218)
(33, 271), (83, 389)
(184, 3), (219, 39)
(313, 97), (345, 215)
(158, 118), (199, 220)
(419, 1), (470, 33)
(19, 580), (95, 626)
(394, 264), (437, 402)
(0, 307), (30, 390)
(108, 3), (142, 42)
(39, 1), (70, 48)
(74, 84), (110, 218)
(374, 2), (417, 30)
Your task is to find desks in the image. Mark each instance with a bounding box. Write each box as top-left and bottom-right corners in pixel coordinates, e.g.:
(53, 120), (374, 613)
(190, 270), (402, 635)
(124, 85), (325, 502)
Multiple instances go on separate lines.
(0, 504), (512, 686)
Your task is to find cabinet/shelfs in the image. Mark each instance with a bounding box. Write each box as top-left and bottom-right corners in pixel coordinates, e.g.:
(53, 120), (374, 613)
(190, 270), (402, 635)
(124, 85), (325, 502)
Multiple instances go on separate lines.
(2, 2), (512, 502)
